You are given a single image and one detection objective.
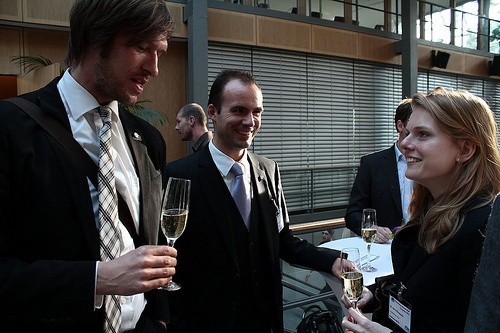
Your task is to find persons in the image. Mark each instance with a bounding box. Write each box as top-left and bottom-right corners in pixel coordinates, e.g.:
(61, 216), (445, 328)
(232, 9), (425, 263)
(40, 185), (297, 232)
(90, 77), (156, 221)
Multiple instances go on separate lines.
(175, 103), (213, 153)
(341, 88), (500, 333)
(0, 0), (177, 333)
(344, 99), (417, 330)
(163, 70), (356, 333)
(319, 229), (334, 245)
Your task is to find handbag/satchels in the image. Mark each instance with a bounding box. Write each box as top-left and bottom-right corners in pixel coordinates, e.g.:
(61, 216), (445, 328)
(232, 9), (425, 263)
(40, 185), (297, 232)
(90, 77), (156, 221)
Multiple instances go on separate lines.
(297, 305), (344, 333)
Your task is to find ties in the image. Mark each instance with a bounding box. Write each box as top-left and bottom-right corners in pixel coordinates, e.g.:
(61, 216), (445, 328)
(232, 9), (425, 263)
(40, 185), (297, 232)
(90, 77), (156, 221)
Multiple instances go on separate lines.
(228, 162), (252, 232)
(97, 106), (122, 333)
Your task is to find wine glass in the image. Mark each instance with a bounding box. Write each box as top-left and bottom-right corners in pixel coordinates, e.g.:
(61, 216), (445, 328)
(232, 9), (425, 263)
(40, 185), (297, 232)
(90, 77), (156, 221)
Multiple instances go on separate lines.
(340, 248), (365, 326)
(360, 209), (377, 272)
(156, 177), (191, 290)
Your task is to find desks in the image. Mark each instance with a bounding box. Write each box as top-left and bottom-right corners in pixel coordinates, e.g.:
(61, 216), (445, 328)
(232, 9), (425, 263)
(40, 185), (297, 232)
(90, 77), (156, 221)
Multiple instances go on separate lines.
(316, 238), (394, 321)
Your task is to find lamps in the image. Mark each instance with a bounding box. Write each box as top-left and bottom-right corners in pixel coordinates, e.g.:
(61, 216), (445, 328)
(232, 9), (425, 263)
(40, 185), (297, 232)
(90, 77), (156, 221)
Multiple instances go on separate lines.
(432, 50), (450, 69)
(488, 54), (500, 76)
